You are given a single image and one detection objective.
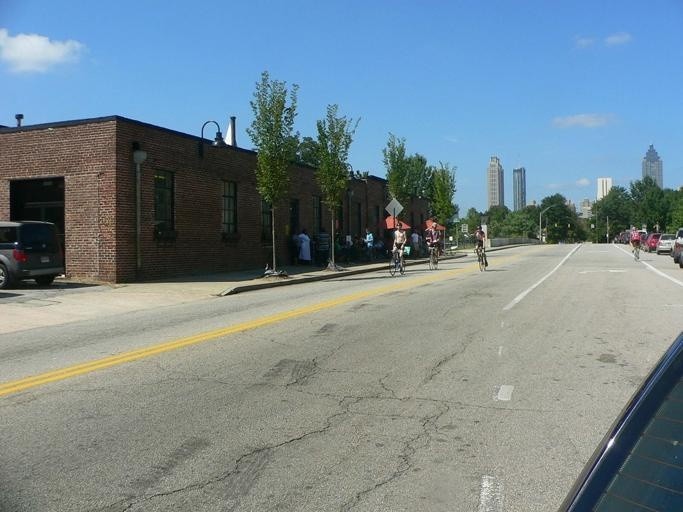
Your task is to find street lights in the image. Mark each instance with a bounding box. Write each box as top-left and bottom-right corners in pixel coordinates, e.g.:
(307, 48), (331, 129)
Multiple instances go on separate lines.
(539, 204), (557, 241)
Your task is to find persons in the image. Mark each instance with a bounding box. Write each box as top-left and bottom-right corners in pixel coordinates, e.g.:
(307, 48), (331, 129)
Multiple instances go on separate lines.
(298, 229), (312, 265)
(474, 225), (488, 266)
(335, 229), (385, 262)
(630, 227), (640, 252)
(392, 222), (442, 273)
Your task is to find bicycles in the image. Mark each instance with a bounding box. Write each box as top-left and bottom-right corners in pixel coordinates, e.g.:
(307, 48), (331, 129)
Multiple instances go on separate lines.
(474, 246), (487, 271)
(391, 254), (405, 276)
(427, 242), (438, 270)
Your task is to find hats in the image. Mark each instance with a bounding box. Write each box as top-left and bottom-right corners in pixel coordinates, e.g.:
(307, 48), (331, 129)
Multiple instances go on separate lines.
(432, 223), (437, 227)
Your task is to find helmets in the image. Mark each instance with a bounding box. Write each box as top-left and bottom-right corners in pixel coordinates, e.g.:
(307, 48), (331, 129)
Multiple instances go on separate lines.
(477, 226), (482, 229)
(397, 223), (402, 226)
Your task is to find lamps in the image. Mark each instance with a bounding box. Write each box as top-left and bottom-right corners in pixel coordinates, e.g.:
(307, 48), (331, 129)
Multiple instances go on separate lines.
(344, 163), (355, 182)
(199, 120), (225, 160)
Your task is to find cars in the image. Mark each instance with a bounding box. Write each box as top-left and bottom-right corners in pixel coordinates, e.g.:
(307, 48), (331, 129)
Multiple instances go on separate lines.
(1, 215), (65, 290)
(614, 228), (683, 268)
